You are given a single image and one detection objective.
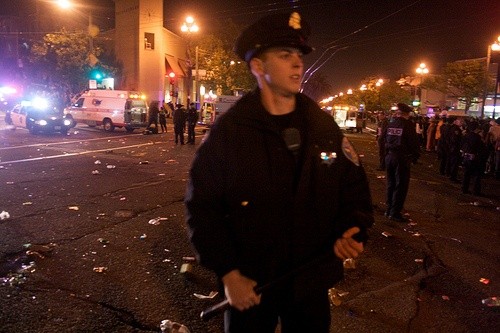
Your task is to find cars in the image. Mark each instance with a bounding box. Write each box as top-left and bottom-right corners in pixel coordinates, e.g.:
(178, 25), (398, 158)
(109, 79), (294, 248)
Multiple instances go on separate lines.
(5, 100), (73, 135)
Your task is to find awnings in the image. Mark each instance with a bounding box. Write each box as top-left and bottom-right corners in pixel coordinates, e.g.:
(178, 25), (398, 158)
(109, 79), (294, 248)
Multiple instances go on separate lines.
(165, 53), (191, 79)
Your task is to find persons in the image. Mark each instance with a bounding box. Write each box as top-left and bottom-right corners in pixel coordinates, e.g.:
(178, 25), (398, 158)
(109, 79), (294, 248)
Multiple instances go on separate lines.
(183, 10), (374, 333)
(30, 87), (198, 145)
(368, 102), (500, 222)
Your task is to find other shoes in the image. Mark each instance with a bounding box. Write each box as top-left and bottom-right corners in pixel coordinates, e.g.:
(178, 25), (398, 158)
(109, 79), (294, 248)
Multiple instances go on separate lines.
(474, 192), (484, 196)
(450, 178), (460, 183)
(463, 190), (472, 194)
(384, 212), (409, 223)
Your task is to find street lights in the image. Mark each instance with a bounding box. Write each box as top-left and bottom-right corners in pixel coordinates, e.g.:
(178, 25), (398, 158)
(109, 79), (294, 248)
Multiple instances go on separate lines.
(376, 78), (385, 109)
(319, 94), (338, 107)
(491, 34), (500, 118)
(360, 84), (367, 105)
(346, 88), (353, 103)
(339, 91), (344, 103)
(415, 61), (429, 112)
(179, 13), (200, 110)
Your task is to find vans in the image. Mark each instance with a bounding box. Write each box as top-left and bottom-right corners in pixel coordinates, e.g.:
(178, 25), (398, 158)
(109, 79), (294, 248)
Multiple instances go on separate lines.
(330, 103), (369, 131)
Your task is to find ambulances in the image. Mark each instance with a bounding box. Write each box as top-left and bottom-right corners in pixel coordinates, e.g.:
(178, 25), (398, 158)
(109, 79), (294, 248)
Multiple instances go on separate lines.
(63, 89), (150, 132)
(197, 95), (243, 127)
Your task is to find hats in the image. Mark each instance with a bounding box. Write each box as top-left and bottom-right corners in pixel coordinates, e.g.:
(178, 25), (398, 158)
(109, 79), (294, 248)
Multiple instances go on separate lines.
(233, 10), (313, 63)
(397, 103), (411, 112)
